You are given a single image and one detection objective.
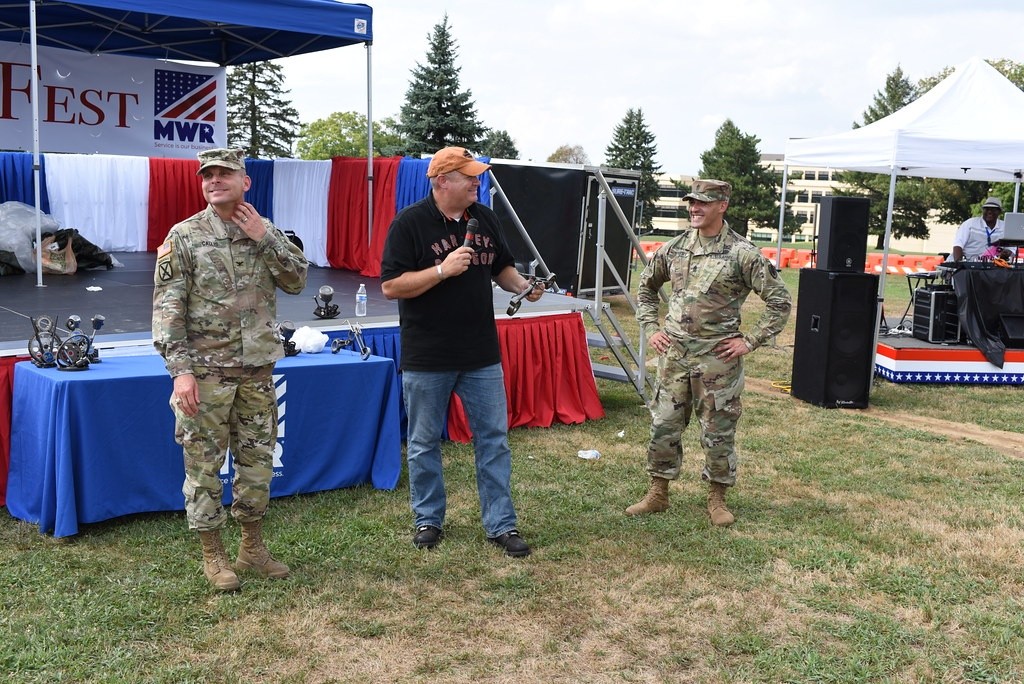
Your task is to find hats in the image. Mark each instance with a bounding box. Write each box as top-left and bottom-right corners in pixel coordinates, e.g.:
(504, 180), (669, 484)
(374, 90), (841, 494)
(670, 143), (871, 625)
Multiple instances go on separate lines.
(682, 179), (732, 203)
(425, 146), (492, 178)
(981, 197), (1000, 208)
(196, 148), (245, 176)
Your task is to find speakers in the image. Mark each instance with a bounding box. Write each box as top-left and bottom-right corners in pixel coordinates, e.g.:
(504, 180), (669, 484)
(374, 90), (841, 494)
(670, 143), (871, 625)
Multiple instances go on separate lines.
(792, 196), (880, 410)
(998, 313), (1024, 349)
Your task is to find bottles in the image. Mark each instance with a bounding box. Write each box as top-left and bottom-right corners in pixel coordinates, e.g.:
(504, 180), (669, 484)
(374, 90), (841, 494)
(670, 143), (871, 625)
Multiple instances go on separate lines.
(355, 283), (367, 316)
(578, 450), (601, 460)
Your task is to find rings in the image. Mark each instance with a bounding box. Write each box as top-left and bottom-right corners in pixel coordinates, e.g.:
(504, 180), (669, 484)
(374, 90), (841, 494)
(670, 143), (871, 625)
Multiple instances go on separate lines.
(538, 295), (542, 299)
(243, 217), (248, 222)
(175, 399), (181, 404)
(726, 350), (731, 355)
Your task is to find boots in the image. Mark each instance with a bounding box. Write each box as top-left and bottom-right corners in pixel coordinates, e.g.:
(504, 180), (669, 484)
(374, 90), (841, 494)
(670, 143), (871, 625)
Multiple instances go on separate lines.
(707, 483), (734, 527)
(236, 525), (290, 579)
(625, 477), (670, 515)
(198, 530), (240, 591)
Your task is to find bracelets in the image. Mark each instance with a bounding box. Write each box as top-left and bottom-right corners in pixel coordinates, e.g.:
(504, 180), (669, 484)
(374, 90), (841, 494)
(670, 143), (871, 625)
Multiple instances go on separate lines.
(742, 337), (753, 351)
(437, 265), (444, 281)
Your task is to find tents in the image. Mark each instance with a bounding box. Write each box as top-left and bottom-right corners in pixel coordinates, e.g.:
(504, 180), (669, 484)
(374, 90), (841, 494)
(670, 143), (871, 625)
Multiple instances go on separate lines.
(773, 52), (1024, 396)
(0, 0), (374, 288)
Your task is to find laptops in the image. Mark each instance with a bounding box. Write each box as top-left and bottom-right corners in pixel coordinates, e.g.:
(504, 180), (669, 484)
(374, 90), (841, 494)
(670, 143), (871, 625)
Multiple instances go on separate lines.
(991, 212), (1024, 245)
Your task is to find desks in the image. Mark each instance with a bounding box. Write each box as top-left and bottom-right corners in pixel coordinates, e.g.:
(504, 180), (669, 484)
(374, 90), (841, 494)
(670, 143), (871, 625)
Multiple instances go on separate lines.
(941, 254), (1024, 348)
(16, 347), (397, 525)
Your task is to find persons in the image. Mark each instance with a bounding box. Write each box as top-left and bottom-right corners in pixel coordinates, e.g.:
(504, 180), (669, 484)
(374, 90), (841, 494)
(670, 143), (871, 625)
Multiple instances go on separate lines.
(151, 144), (312, 592)
(941, 196), (1018, 285)
(624, 180), (793, 526)
(379, 147), (546, 557)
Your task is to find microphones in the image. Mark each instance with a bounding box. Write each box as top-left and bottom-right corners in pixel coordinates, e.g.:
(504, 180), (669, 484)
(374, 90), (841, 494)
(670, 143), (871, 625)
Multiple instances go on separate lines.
(464, 218), (478, 248)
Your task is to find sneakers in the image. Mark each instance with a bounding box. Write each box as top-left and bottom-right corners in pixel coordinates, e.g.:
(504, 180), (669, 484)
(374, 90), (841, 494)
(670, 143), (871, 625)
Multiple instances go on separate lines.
(413, 525), (442, 549)
(488, 530), (531, 557)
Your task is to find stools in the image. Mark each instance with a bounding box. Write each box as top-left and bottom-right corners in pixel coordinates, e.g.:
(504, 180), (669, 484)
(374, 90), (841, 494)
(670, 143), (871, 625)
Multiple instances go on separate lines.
(901, 268), (941, 338)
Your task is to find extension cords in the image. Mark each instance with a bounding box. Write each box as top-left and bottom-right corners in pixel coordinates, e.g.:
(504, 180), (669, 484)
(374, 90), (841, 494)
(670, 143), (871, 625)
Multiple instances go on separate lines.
(890, 329), (911, 335)
